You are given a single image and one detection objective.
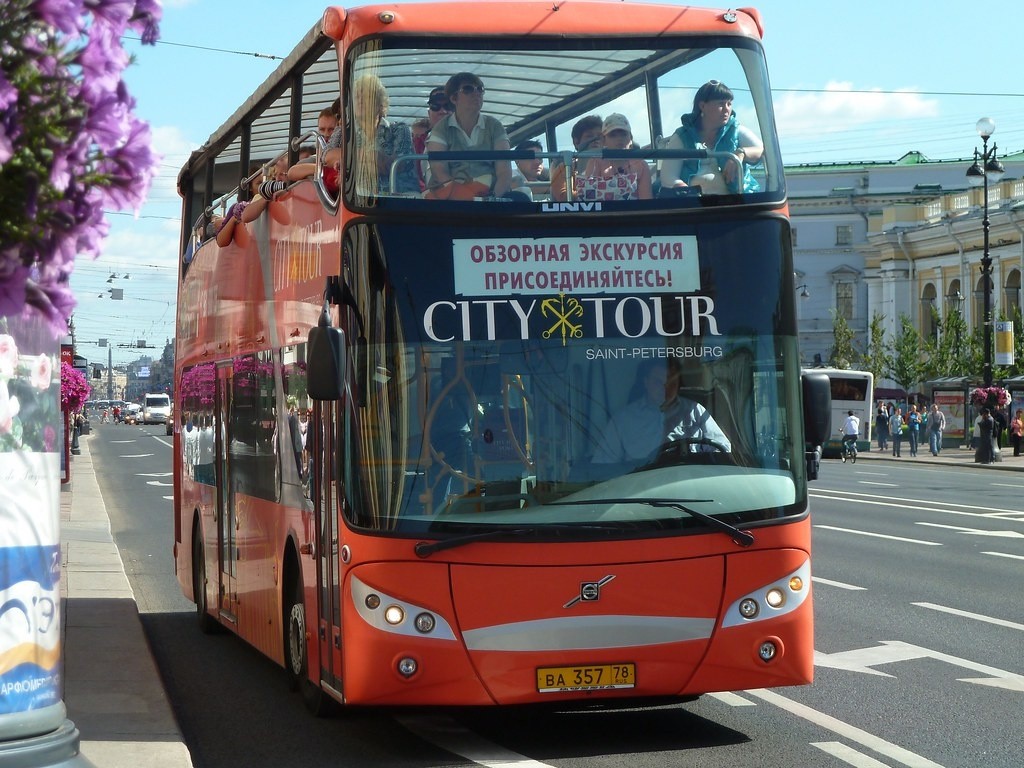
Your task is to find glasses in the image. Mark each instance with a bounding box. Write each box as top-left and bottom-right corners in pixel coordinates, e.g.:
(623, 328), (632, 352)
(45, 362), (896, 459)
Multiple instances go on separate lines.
(412, 133), (420, 137)
(337, 114), (341, 121)
(448, 85), (485, 95)
(430, 102), (455, 112)
(704, 79), (721, 94)
(272, 172), (287, 177)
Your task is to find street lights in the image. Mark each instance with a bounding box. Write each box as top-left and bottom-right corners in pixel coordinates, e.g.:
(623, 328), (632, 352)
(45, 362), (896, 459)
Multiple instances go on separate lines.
(964, 115), (1007, 462)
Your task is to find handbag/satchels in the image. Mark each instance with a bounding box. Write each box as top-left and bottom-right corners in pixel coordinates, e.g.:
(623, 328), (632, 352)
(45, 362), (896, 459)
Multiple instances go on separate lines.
(1009, 428), (1014, 433)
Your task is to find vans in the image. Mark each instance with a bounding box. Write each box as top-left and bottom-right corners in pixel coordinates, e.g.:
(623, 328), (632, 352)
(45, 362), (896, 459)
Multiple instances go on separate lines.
(142, 392), (171, 425)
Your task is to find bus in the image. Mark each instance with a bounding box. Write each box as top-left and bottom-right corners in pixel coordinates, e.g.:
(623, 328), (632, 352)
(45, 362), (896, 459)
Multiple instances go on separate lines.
(800, 366), (876, 452)
(165, 3), (835, 715)
(86, 399), (125, 410)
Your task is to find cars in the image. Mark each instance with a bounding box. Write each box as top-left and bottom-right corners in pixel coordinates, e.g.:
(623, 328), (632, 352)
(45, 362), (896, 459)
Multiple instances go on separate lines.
(135, 407), (143, 425)
(166, 407), (174, 436)
(120, 410), (136, 425)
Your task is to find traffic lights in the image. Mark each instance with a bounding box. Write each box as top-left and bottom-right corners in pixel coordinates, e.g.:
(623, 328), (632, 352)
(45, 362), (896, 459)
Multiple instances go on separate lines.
(168, 382), (171, 386)
(165, 386), (169, 390)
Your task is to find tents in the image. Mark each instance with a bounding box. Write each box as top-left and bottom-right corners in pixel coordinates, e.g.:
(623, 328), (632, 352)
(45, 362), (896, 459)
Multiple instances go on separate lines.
(873, 388), (931, 403)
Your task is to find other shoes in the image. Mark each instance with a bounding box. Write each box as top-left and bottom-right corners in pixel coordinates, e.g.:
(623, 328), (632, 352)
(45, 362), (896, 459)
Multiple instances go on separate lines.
(981, 456), (996, 464)
(909, 451), (917, 457)
(897, 454), (901, 457)
(893, 452), (896, 456)
(1014, 454), (1021, 457)
(880, 447), (888, 451)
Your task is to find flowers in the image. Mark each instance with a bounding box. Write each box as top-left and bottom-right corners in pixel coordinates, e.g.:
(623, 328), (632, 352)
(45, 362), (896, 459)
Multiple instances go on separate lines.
(179, 357), (310, 407)
(0, 1), (166, 452)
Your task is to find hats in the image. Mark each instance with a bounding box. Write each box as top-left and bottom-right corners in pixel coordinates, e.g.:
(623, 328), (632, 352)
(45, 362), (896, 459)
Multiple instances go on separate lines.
(878, 409), (884, 414)
(601, 113), (631, 136)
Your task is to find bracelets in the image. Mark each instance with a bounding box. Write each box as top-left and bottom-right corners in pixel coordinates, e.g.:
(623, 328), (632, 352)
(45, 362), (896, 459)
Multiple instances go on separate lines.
(736, 146), (747, 157)
(235, 219), (238, 224)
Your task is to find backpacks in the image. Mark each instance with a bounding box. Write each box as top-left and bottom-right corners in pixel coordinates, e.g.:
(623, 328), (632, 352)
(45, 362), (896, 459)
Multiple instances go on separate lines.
(992, 420), (1000, 438)
(929, 412), (942, 432)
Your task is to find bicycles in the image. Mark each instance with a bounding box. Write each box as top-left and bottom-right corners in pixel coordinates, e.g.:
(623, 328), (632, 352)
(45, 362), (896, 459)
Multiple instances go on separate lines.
(112, 414), (120, 425)
(838, 428), (858, 464)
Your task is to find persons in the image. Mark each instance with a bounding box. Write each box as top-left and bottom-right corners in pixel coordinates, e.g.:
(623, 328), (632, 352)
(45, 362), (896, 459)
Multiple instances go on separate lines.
(588, 353), (734, 461)
(183, 71), (763, 197)
(87, 405), (121, 424)
(841, 400), (1024, 461)
(181, 403), (335, 502)
(355, 363), (461, 509)
